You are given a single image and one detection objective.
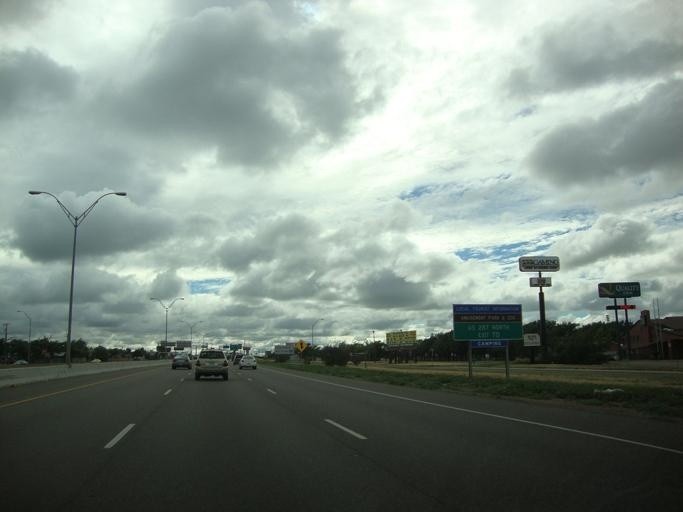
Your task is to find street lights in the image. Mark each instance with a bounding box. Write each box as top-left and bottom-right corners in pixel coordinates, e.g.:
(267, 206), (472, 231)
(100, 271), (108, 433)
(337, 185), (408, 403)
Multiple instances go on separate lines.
(312, 318), (324, 346)
(28, 190), (127, 364)
(16, 310), (32, 357)
(150, 297), (184, 361)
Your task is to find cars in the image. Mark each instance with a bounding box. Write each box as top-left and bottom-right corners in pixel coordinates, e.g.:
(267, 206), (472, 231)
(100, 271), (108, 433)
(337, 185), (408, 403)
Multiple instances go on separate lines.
(233, 354), (257, 369)
(194, 350), (228, 381)
(172, 355), (192, 369)
